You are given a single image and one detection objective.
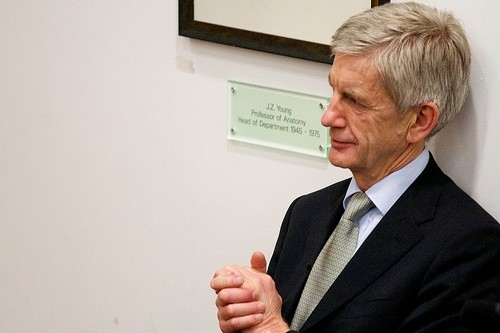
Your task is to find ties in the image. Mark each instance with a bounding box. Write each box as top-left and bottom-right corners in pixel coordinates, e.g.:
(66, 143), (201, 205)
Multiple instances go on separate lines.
(290, 192), (376, 330)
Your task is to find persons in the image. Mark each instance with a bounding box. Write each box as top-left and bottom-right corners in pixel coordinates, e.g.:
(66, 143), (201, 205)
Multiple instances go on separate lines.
(211, 3), (500, 333)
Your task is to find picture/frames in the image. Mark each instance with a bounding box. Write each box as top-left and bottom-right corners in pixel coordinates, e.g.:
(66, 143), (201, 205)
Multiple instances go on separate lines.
(178, 0), (391, 65)
